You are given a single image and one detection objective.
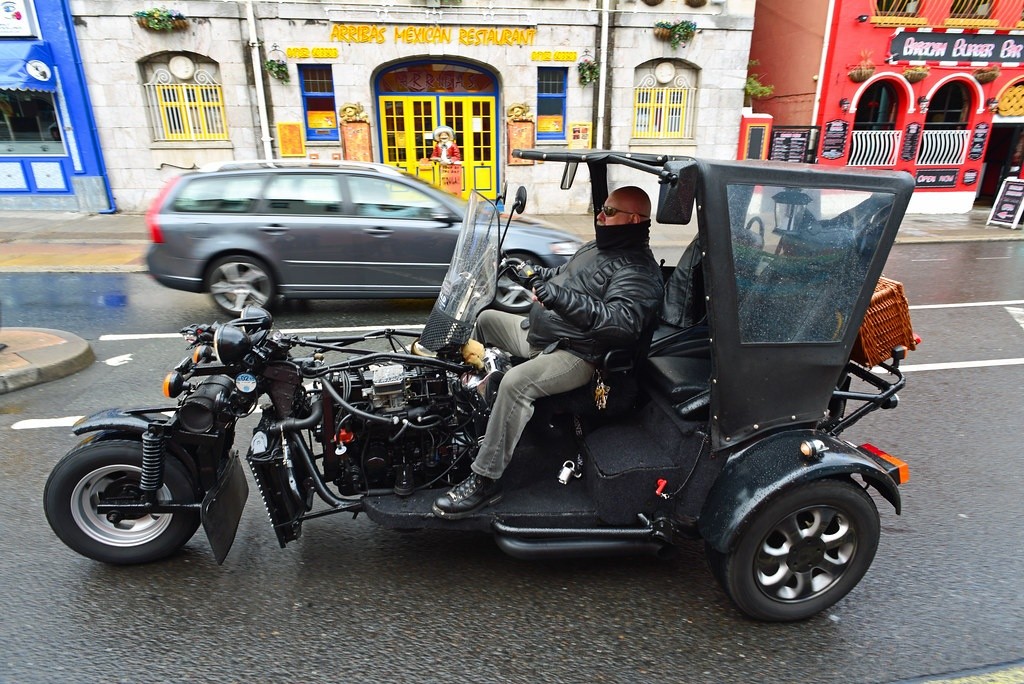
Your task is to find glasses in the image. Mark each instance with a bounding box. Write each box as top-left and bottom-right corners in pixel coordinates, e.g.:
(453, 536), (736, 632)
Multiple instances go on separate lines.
(601, 205), (647, 217)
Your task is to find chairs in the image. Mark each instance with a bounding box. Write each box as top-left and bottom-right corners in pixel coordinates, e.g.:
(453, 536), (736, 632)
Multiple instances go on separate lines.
(521, 216), (858, 419)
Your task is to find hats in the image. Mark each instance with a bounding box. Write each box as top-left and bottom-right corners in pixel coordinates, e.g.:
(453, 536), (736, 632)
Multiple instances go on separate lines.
(433, 125), (454, 141)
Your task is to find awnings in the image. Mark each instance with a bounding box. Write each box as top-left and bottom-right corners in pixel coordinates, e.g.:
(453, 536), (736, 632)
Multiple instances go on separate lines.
(0, 39), (58, 92)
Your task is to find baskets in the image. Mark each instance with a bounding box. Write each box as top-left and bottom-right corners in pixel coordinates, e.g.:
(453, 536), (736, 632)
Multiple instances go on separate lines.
(859, 276), (916, 367)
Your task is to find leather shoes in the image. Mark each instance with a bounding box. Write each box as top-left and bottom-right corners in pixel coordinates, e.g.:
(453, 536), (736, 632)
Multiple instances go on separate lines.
(432, 471), (504, 519)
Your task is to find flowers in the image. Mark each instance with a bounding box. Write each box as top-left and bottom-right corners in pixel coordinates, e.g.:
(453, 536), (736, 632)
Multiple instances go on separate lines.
(654, 18), (697, 50)
(578, 59), (600, 88)
(133, 7), (185, 32)
(265, 59), (290, 85)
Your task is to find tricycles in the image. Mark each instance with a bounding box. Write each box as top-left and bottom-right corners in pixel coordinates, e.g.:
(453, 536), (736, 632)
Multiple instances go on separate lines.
(42, 148), (924, 624)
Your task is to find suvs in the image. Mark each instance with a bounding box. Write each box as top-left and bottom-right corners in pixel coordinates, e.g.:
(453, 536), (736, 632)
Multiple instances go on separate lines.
(141, 153), (593, 324)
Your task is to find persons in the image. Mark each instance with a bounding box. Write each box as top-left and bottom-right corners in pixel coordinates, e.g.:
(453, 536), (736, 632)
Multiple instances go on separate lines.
(429, 184), (668, 520)
(429, 124), (460, 168)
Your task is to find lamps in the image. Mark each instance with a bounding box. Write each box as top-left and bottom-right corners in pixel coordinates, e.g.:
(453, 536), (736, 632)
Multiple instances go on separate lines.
(917, 95), (929, 115)
(839, 97), (850, 113)
(987, 97), (998, 114)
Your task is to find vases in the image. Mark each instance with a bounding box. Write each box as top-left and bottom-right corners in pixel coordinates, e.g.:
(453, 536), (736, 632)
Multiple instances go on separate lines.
(654, 27), (695, 43)
(137, 18), (190, 33)
